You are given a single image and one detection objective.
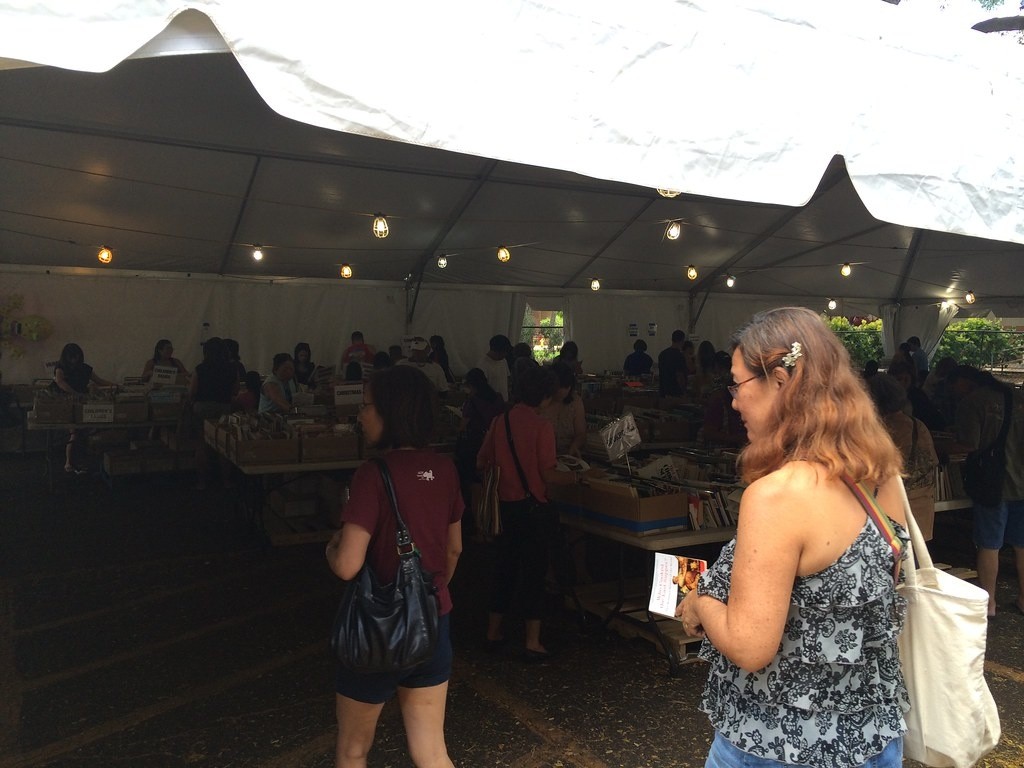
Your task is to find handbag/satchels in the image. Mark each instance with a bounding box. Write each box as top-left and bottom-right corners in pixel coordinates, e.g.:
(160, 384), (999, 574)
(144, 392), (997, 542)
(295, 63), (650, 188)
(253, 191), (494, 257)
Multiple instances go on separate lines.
(962, 442), (1007, 506)
(525, 493), (560, 542)
(330, 456), (441, 675)
(475, 414), (504, 542)
(893, 470), (1002, 768)
(292, 383), (314, 405)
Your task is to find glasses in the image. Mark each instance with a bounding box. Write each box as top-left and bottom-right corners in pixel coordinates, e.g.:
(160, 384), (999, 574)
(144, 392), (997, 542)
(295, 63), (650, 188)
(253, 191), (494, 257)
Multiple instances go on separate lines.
(358, 398), (375, 410)
(728, 364), (777, 401)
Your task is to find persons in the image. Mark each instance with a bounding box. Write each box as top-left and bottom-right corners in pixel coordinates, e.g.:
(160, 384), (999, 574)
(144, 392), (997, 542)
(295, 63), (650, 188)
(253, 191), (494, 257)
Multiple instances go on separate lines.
(258, 353), (296, 411)
(890, 354), (935, 432)
(186, 336), (261, 490)
(898, 337), (928, 379)
(861, 360), (878, 378)
(623, 329), (748, 446)
(326, 367), (464, 768)
(952, 365), (1024, 619)
(375, 337), (447, 394)
(429, 335), (449, 369)
(922, 357), (957, 404)
(144, 339), (189, 380)
(459, 334), (586, 658)
(54, 344), (115, 473)
(676, 306), (913, 768)
(294, 343), (317, 389)
(871, 373), (935, 541)
(340, 330), (379, 364)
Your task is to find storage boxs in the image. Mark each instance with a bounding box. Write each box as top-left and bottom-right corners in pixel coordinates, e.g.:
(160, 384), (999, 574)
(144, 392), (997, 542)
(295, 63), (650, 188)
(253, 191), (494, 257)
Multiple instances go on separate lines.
(581, 370), (711, 446)
(560, 483), (689, 534)
(30, 380), (190, 421)
(203, 409), (359, 466)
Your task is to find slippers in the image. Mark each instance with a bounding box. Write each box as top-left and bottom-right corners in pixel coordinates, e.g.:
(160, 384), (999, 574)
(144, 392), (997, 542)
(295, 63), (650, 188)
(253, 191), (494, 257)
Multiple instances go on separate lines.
(1011, 599), (1024, 616)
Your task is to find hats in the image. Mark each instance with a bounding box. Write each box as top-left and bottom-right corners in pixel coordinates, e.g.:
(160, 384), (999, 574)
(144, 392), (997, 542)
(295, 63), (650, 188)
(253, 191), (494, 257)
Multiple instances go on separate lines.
(411, 340), (427, 350)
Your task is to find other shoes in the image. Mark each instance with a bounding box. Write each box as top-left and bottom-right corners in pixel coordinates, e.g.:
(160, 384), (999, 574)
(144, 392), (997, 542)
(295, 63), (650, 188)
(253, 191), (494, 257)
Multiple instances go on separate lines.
(486, 637), (506, 650)
(525, 648), (549, 661)
(63, 463), (73, 473)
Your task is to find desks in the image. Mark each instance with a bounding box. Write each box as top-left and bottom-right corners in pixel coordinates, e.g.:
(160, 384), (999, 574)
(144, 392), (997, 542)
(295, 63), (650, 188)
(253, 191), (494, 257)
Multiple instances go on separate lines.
(227, 449), (472, 547)
(27, 411), (192, 493)
(555, 499), (978, 680)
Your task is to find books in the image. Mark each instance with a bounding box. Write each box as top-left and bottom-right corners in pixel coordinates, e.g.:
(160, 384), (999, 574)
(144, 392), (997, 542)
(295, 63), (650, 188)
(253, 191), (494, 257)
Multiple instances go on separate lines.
(443, 366), (455, 383)
(936, 462), (963, 502)
(445, 400), (466, 424)
(585, 368), (743, 532)
(215, 366), (362, 440)
(87, 367), (185, 404)
(648, 552), (707, 622)
(556, 455), (588, 471)
